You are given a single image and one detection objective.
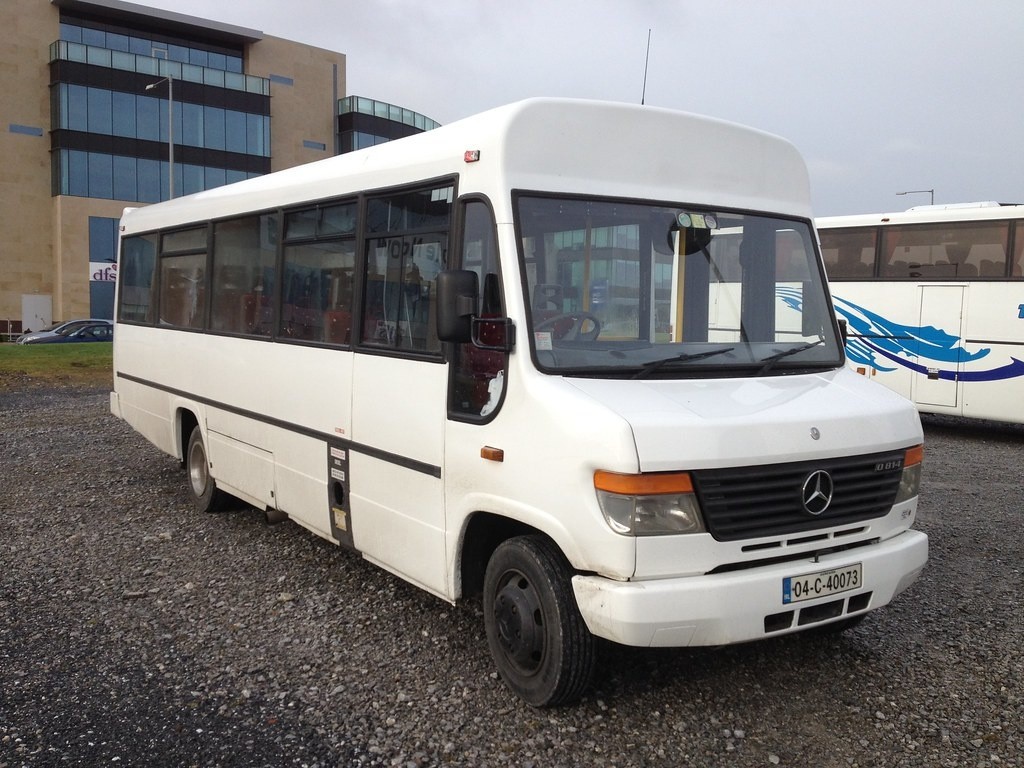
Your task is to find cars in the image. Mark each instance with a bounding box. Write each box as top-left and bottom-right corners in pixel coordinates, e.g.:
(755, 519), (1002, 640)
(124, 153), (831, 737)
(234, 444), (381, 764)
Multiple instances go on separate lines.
(16, 318), (113, 345)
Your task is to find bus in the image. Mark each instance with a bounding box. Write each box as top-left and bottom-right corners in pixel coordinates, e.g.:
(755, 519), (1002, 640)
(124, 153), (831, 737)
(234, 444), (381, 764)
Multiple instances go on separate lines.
(109, 97), (928, 710)
(669, 198), (1024, 425)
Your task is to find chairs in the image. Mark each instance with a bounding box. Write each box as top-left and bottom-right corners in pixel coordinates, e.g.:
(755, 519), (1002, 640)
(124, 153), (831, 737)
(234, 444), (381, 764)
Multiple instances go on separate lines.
(146, 267), (576, 406)
